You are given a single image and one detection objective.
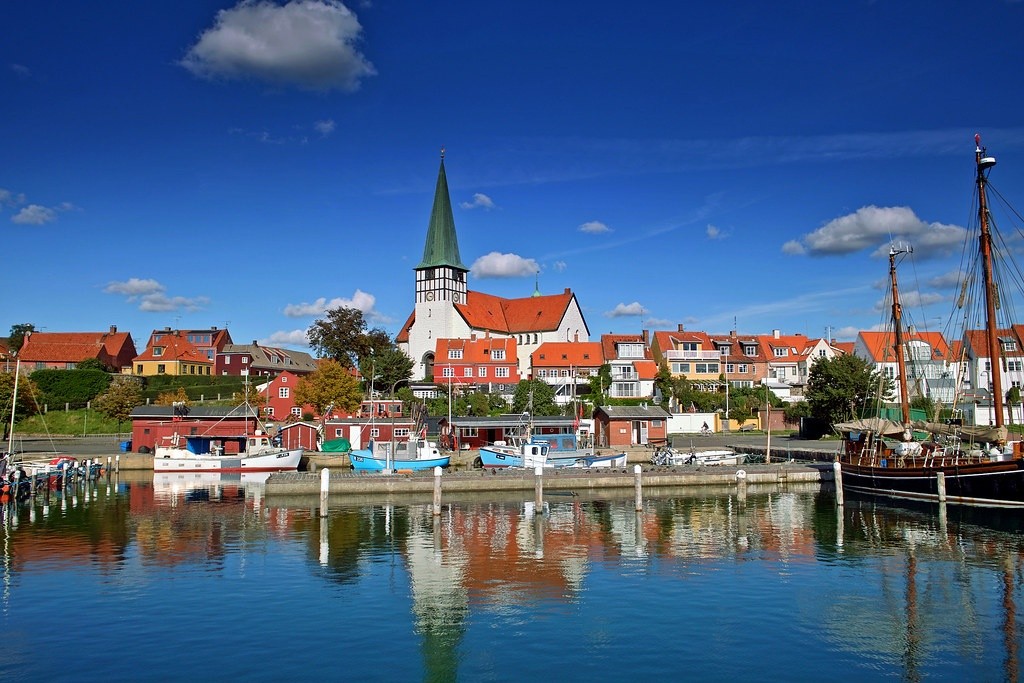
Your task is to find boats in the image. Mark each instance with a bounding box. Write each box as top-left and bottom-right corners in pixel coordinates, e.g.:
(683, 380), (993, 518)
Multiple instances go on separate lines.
(1, 360), (79, 503)
(347, 435), (451, 471)
(152, 472), (272, 515)
(153, 366), (304, 472)
(478, 390), (627, 469)
(650, 439), (748, 465)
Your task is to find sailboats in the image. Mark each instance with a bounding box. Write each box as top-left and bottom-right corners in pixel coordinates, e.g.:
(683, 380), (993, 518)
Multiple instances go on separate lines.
(804, 131), (1024, 529)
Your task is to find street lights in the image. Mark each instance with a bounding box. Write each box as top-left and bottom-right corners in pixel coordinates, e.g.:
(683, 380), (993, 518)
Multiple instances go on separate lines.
(370, 374), (383, 420)
(765, 356), (781, 432)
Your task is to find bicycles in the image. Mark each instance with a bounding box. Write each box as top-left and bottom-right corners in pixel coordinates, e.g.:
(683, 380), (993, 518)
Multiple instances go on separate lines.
(698, 428), (714, 437)
